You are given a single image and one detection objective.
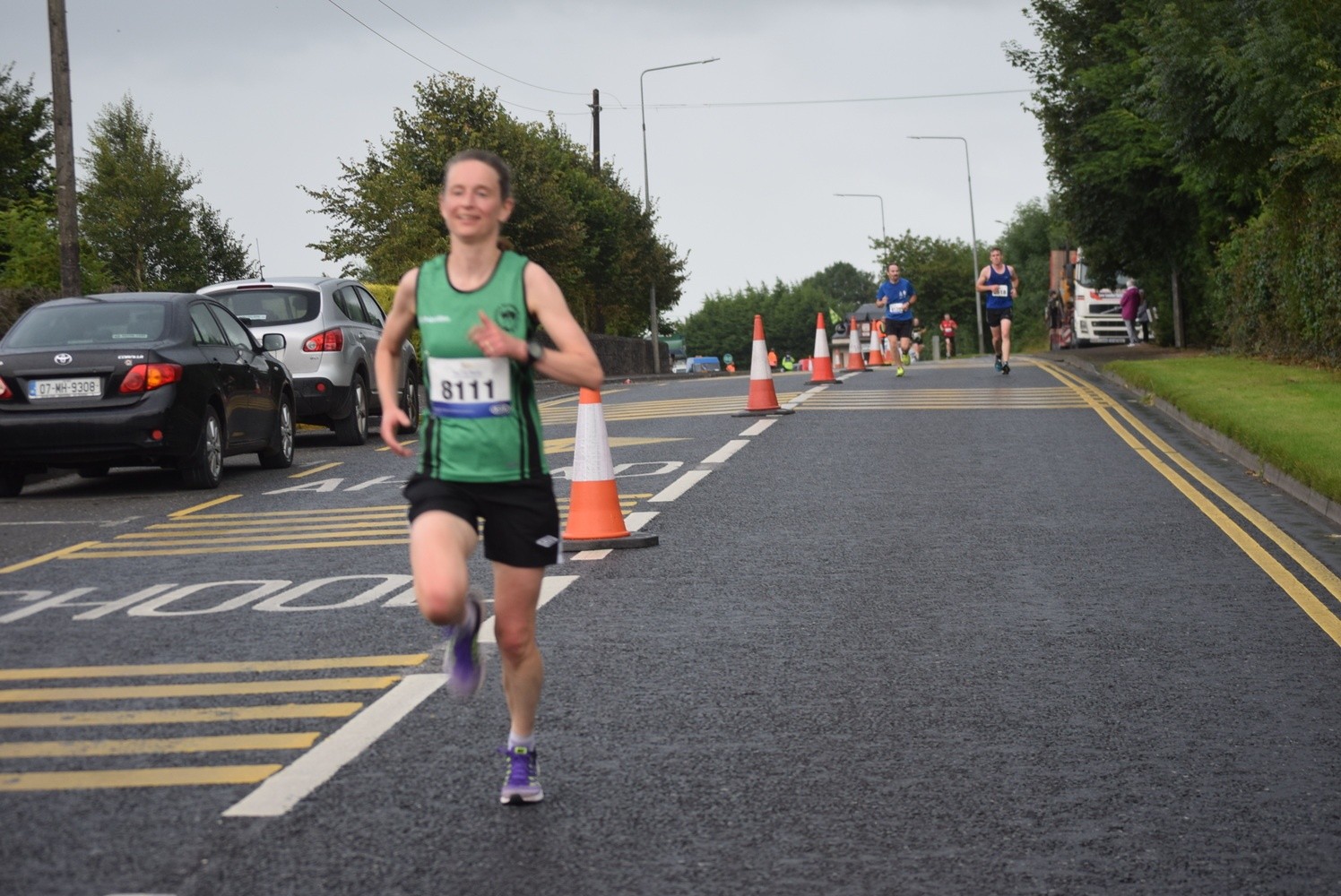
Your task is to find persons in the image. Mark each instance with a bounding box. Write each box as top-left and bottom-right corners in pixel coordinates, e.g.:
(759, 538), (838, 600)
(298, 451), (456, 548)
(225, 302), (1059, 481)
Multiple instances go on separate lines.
(875, 263), (917, 377)
(373, 148), (604, 805)
(768, 348), (777, 370)
(725, 362), (735, 372)
(975, 247), (1020, 374)
(878, 315), (926, 361)
(781, 352), (794, 371)
(1120, 279), (1148, 347)
(938, 313), (957, 359)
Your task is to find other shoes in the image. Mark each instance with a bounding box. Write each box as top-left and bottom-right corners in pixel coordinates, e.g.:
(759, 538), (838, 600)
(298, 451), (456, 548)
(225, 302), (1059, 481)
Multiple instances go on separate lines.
(1126, 343), (1135, 347)
(1003, 364), (1010, 375)
(995, 354), (1002, 371)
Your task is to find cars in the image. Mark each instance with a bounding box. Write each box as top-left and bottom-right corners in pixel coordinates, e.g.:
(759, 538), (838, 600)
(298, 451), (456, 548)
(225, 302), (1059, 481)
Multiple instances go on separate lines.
(0, 287), (299, 501)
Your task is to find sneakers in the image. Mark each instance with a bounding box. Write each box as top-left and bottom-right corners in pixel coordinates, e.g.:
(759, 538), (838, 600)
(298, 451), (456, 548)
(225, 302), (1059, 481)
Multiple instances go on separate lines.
(896, 367), (904, 376)
(900, 353), (911, 366)
(496, 745), (544, 805)
(443, 599), (486, 696)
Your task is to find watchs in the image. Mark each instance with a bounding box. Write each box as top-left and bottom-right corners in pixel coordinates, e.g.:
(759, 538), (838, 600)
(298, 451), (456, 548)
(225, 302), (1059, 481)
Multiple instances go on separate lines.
(521, 342), (543, 366)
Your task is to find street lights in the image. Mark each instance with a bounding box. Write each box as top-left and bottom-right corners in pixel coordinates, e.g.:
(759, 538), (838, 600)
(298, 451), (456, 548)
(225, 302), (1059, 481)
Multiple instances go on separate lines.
(639, 57), (722, 376)
(832, 193), (889, 280)
(904, 135), (986, 355)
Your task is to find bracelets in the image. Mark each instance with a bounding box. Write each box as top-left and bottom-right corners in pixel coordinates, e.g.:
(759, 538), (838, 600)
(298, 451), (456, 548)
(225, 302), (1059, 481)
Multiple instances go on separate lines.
(909, 301), (912, 304)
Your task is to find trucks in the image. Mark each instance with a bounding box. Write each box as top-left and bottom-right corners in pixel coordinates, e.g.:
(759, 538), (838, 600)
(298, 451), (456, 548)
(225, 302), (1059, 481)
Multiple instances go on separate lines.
(1044, 244), (1158, 349)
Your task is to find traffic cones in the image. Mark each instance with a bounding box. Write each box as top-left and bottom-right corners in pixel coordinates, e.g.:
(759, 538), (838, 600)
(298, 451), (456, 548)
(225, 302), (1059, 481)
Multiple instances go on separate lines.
(839, 314), (874, 374)
(557, 384), (659, 552)
(731, 314), (795, 418)
(802, 312), (843, 384)
(865, 318), (894, 367)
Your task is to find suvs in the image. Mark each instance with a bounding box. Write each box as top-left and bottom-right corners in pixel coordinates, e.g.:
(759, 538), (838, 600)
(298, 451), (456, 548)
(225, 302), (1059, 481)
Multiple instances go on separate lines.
(186, 274), (421, 447)
